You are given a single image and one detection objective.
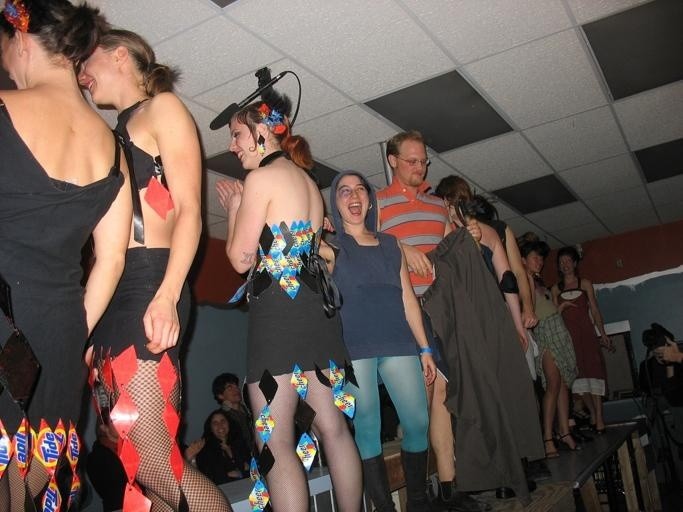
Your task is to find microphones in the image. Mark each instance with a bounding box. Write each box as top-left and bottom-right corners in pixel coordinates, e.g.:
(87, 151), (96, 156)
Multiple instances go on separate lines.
(209, 70), (288, 131)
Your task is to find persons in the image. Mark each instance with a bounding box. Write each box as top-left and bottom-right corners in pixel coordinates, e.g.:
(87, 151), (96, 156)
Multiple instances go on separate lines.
(637, 336), (683, 407)
(0, 1), (230, 511)
(199, 94), (615, 510)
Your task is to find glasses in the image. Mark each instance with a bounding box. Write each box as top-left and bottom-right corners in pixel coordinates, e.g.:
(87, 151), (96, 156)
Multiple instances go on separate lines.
(400, 158), (429, 166)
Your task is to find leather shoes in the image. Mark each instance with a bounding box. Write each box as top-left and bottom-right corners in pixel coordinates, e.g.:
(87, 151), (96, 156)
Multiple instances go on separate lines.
(361, 420), (604, 512)
